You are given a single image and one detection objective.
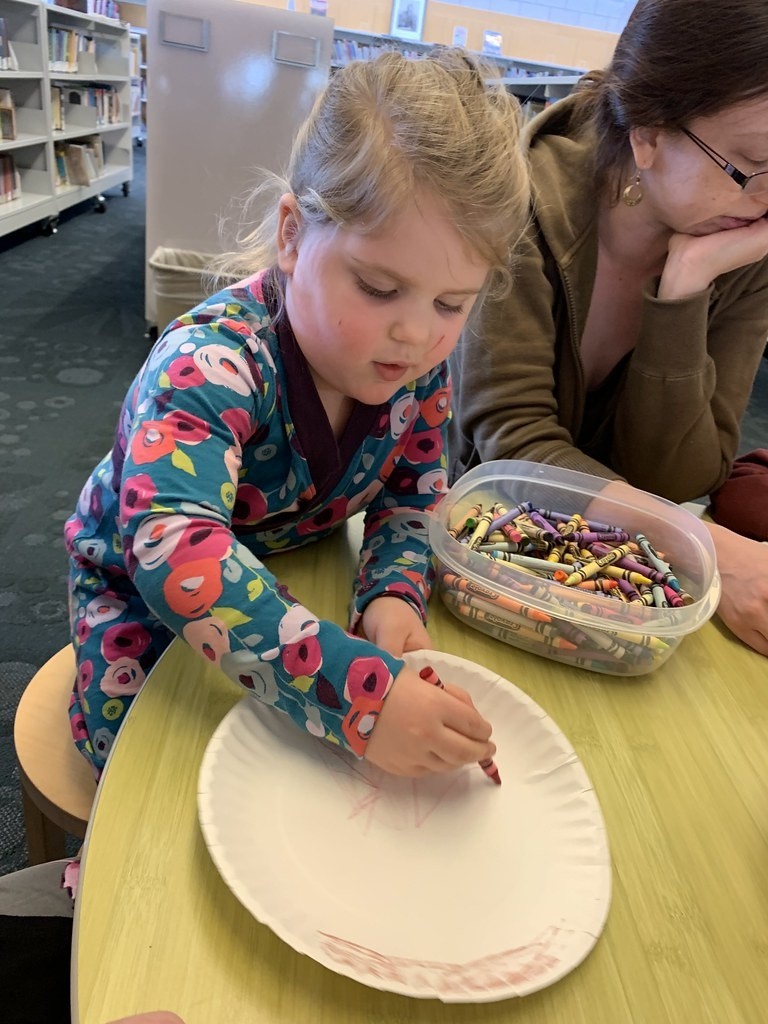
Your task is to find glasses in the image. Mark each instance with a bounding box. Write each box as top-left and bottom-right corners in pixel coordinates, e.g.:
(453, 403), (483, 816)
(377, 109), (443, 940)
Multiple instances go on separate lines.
(668, 121), (768, 196)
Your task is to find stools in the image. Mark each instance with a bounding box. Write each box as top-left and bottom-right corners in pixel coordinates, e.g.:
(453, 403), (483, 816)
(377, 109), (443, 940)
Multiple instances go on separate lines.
(12, 637), (105, 864)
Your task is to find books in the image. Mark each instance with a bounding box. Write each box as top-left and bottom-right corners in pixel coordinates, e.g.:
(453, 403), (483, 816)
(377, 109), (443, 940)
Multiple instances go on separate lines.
(49, 81), (123, 132)
(129, 35), (148, 137)
(45, 0), (120, 21)
(0, 17), (22, 73)
(53, 133), (106, 196)
(0, 88), (17, 141)
(330, 40), (431, 72)
(480, 62), (568, 132)
(46, 26), (100, 77)
(0, 153), (22, 204)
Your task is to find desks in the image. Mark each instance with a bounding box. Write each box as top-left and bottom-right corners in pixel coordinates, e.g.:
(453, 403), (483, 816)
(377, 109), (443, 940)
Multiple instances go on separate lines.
(71, 502), (768, 1024)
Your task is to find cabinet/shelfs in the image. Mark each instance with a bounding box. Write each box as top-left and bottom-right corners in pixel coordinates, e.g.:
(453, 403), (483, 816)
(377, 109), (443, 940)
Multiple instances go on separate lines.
(0, 0), (146, 239)
(329, 26), (590, 127)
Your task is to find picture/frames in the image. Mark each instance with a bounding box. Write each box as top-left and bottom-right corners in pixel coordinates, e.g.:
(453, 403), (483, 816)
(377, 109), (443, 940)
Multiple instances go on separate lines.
(389, 0), (427, 41)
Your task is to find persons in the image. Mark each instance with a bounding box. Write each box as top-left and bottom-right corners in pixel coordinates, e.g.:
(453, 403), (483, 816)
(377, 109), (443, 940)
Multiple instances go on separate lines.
(64, 48), (537, 776)
(448, 0), (768, 662)
